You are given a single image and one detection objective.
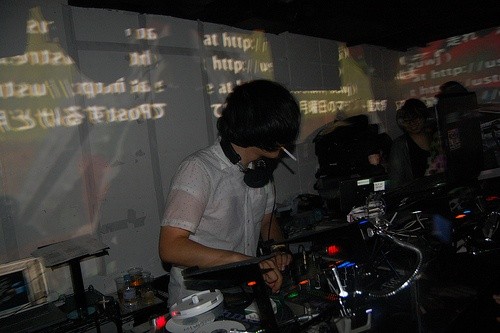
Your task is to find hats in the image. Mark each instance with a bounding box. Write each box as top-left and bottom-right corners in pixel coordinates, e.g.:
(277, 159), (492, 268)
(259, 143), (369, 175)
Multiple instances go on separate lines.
(397, 99), (429, 109)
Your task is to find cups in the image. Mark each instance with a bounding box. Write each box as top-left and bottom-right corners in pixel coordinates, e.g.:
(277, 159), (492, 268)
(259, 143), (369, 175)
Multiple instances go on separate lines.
(113, 266), (156, 308)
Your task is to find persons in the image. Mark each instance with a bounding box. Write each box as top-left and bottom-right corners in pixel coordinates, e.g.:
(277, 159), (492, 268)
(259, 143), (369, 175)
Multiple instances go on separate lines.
(158, 78), (302, 293)
(392, 98), (440, 176)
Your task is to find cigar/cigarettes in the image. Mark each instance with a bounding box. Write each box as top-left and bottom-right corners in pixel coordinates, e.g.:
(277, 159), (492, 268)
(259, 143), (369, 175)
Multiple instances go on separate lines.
(280, 146), (298, 161)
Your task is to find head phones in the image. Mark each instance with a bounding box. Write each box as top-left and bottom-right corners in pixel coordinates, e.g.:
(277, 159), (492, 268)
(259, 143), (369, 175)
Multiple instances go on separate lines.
(220, 137), (275, 187)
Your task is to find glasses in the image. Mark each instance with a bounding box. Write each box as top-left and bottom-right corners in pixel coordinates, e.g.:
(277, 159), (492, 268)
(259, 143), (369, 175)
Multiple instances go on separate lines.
(404, 117), (420, 124)
